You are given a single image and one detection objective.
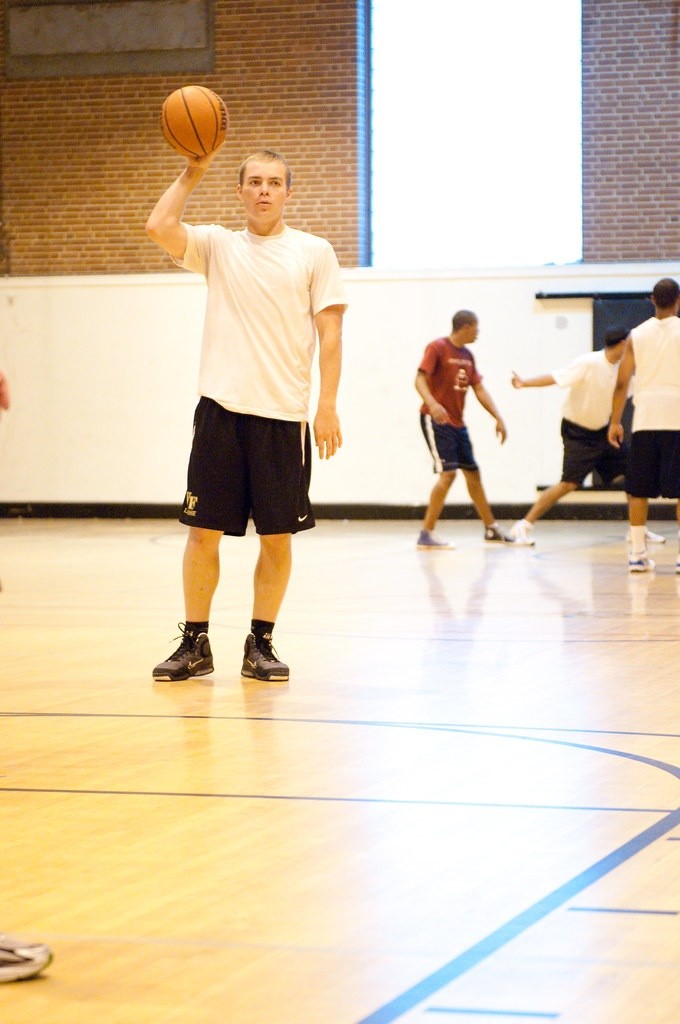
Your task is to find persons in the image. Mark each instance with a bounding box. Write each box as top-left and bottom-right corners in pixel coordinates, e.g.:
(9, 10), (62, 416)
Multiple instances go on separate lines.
(508, 325), (666, 546)
(608, 278), (680, 574)
(145, 140), (349, 682)
(0, 368), (11, 411)
(415, 310), (515, 549)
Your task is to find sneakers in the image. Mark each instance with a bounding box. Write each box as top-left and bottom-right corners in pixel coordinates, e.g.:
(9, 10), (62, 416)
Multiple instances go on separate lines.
(0, 933), (52, 983)
(484, 527), (514, 543)
(510, 521), (534, 546)
(242, 634), (289, 680)
(626, 527), (666, 544)
(416, 531), (447, 549)
(153, 622), (215, 680)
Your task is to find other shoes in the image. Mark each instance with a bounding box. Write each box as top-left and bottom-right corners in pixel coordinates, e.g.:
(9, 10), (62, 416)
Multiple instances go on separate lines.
(676, 562), (680, 573)
(629, 556), (654, 571)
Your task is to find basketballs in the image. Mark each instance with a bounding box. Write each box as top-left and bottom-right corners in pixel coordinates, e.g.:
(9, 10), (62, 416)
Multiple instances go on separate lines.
(159, 85), (230, 158)
